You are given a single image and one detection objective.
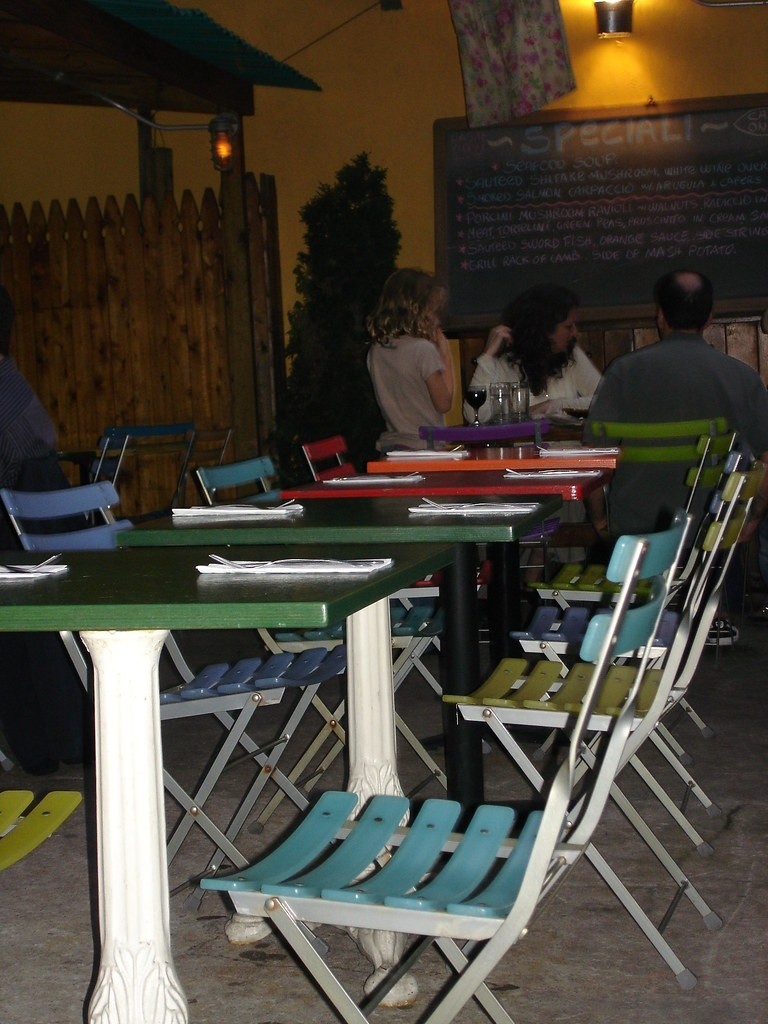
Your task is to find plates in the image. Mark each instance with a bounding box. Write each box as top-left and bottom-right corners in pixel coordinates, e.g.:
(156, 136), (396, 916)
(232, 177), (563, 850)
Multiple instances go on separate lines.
(564, 408), (588, 418)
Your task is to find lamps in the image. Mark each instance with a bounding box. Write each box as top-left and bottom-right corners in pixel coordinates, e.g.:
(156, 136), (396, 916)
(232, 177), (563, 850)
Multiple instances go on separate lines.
(53, 75), (237, 171)
(595, 0), (634, 40)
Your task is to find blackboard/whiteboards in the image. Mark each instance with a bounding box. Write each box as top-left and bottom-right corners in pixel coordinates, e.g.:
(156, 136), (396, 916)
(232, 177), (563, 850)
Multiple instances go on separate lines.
(433, 93), (768, 334)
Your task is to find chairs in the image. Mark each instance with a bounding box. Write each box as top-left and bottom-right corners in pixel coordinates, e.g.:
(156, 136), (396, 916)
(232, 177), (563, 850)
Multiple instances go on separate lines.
(1, 401), (767, 1024)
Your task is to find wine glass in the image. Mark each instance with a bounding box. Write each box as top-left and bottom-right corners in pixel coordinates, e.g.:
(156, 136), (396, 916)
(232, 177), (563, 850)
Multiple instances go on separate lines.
(465, 385), (487, 427)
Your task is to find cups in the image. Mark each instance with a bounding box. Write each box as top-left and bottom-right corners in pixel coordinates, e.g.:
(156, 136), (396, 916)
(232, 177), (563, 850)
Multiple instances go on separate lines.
(510, 381), (529, 421)
(489, 382), (510, 423)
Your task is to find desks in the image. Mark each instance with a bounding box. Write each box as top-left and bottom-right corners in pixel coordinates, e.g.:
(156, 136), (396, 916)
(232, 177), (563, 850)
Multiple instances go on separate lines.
(367, 445), (619, 704)
(0, 542), (460, 1024)
(280, 468), (603, 749)
(110, 491), (565, 864)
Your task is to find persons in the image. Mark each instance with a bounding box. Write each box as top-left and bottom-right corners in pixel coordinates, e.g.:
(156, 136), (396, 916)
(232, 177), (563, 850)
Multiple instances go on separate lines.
(581, 269), (768, 549)
(463, 283), (609, 544)
(365, 266), (455, 452)
